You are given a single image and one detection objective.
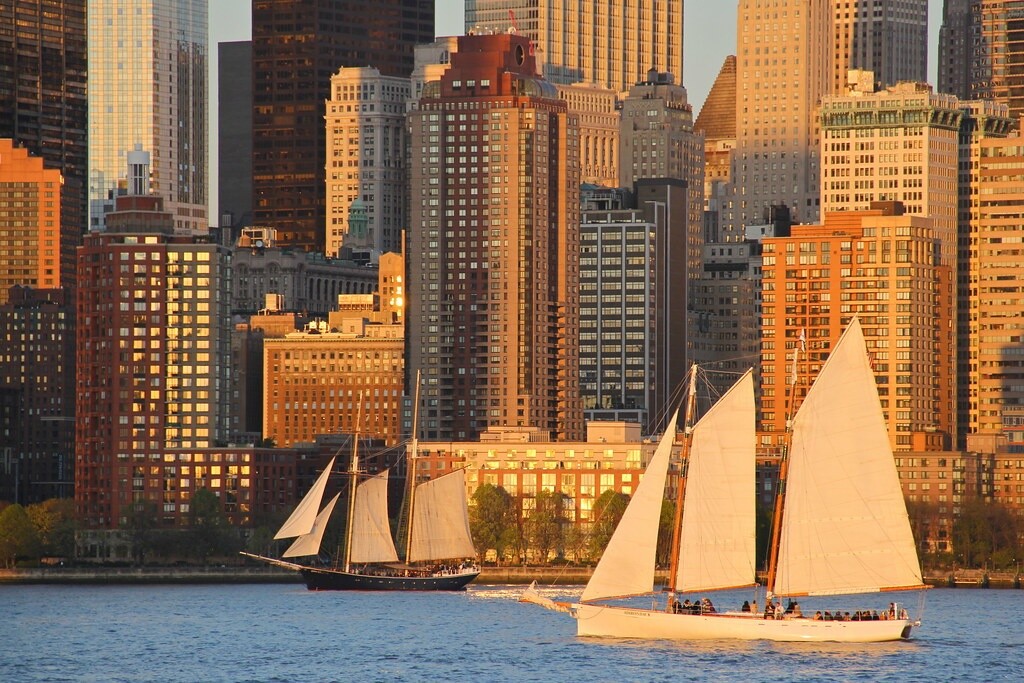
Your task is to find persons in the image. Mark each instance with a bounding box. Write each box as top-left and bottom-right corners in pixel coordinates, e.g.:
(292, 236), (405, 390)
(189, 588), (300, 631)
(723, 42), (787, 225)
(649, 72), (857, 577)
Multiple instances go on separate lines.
(672, 597), (713, 615)
(742, 600), (757, 613)
(813, 603), (895, 622)
(764, 602), (801, 620)
(349, 564), (464, 575)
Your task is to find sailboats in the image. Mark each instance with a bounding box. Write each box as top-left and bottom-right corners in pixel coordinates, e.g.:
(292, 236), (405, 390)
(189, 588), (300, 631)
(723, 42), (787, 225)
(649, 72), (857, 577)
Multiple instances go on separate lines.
(237, 370), (482, 592)
(518, 313), (934, 643)
